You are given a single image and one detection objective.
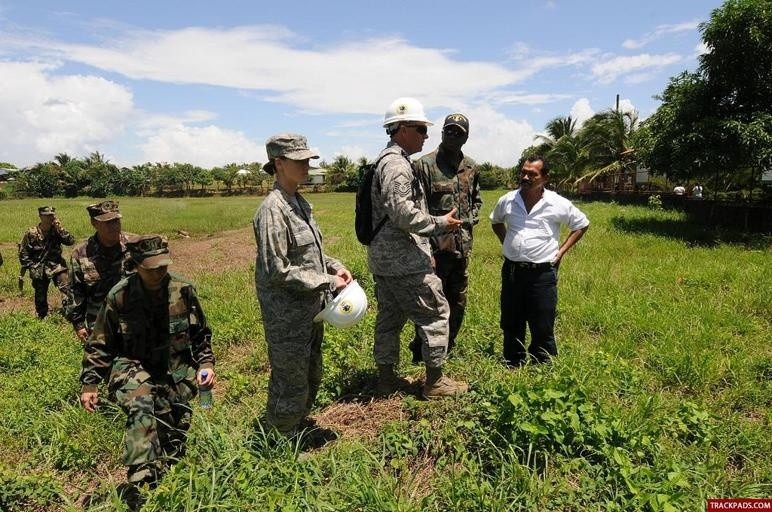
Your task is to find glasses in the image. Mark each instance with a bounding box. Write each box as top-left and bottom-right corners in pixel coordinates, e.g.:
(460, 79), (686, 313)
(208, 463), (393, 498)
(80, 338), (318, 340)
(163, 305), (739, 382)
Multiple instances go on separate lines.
(444, 129), (468, 139)
(399, 124), (428, 136)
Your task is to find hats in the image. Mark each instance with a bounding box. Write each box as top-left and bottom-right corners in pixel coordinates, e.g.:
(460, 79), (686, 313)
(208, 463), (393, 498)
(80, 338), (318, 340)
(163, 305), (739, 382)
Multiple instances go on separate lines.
(125, 233), (174, 269)
(86, 200), (122, 222)
(38, 206), (56, 216)
(442, 114), (470, 134)
(266, 134), (320, 163)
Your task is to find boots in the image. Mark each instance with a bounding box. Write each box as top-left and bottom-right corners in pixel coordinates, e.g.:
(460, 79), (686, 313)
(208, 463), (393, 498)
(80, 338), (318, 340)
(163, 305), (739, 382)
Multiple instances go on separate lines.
(506, 262), (552, 268)
(376, 376), (414, 396)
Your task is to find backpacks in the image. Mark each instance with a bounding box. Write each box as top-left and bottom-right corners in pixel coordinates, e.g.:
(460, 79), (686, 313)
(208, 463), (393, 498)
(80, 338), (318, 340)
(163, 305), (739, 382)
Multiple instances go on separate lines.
(355, 152), (423, 245)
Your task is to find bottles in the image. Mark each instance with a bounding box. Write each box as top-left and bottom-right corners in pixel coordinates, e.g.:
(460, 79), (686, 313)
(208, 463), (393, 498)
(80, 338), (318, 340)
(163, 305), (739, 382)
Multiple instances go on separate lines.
(198, 370), (213, 410)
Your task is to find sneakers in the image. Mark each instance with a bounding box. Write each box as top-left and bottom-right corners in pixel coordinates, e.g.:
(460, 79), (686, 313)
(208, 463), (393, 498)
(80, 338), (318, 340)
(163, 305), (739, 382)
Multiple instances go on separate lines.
(423, 367), (468, 398)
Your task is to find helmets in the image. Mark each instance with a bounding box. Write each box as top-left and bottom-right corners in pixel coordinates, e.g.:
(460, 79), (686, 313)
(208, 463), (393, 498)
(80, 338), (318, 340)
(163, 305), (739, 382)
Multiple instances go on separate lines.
(314, 279), (368, 329)
(382, 98), (434, 132)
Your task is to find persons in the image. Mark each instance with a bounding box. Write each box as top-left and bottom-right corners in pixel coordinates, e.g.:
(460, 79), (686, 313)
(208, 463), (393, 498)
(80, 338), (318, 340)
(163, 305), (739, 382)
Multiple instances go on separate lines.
(79, 234), (216, 511)
(673, 183), (686, 195)
(489, 153), (590, 369)
(18, 206), (75, 319)
(252, 133), (353, 443)
(65, 200), (141, 344)
(367, 98), (468, 399)
(408, 114), (483, 364)
(692, 183), (703, 198)
